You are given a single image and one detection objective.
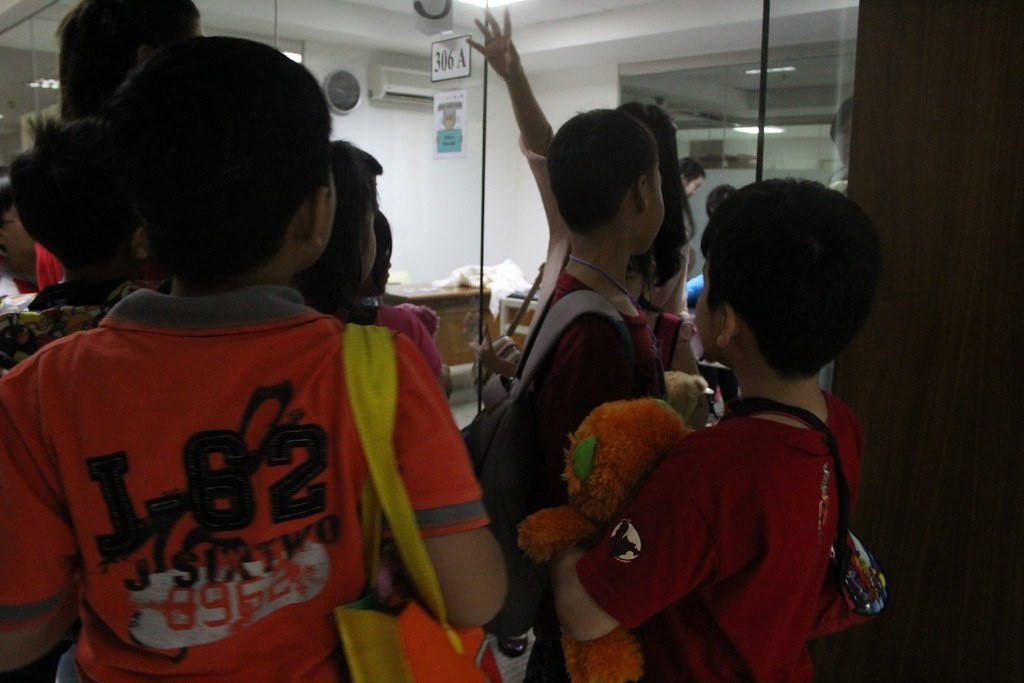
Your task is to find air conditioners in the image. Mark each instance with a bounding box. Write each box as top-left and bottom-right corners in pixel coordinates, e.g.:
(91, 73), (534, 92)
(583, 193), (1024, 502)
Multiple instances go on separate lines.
(367, 64), (463, 108)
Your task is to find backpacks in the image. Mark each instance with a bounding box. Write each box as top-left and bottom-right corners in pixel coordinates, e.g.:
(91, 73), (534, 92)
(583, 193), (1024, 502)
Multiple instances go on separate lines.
(460, 289), (636, 640)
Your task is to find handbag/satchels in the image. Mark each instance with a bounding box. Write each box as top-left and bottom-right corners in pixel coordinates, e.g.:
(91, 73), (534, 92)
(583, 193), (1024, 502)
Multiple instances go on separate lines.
(328, 323), (504, 683)
(805, 526), (896, 642)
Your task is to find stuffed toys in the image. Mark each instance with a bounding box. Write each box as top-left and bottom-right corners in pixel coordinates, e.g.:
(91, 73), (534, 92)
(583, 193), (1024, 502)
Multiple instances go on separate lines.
(517, 398), (695, 683)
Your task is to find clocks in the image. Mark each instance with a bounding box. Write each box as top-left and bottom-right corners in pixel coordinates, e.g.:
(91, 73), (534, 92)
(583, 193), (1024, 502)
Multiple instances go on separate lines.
(322, 69), (362, 114)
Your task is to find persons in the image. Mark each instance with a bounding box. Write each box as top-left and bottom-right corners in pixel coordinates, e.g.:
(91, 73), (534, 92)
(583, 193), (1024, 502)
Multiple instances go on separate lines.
(0, 36), (507, 683)
(468, 8), (736, 683)
(553, 176), (883, 683)
(0, 0), (453, 400)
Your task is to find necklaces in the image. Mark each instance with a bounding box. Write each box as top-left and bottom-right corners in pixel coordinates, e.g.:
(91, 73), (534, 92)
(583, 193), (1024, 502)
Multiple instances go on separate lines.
(569, 255), (657, 352)
